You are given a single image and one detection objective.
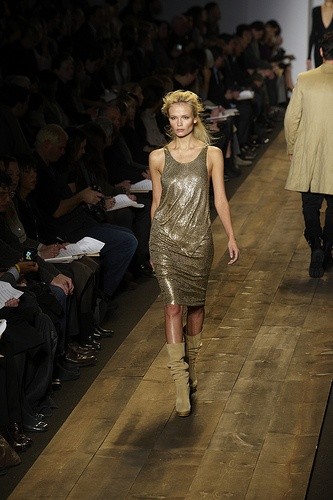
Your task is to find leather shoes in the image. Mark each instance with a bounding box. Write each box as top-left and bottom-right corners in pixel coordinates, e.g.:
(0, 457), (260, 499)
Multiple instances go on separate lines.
(10, 323), (115, 451)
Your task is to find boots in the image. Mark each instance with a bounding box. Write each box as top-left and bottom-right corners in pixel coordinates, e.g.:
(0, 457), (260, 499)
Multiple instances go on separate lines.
(165, 336), (192, 417)
(183, 325), (204, 389)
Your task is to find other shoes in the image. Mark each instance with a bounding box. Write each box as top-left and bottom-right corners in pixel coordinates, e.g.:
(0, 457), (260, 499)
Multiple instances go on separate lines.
(109, 259), (152, 312)
(0, 470), (8, 475)
(309, 241), (333, 278)
(224, 85), (294, 183)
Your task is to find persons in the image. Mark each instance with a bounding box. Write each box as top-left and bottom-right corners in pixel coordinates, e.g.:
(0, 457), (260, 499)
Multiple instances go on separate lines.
(0, 0), (294, 468)
(148, 90), (240, 416)
(284, 32), (333, 279)
(307, 0), (333, 69)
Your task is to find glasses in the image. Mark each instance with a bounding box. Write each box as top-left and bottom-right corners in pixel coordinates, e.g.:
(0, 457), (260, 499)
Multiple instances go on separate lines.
(0, 192), (16, 199)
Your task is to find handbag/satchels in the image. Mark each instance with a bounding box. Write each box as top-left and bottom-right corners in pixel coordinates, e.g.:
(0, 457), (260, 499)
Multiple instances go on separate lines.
(0, 435), (21, 467)
